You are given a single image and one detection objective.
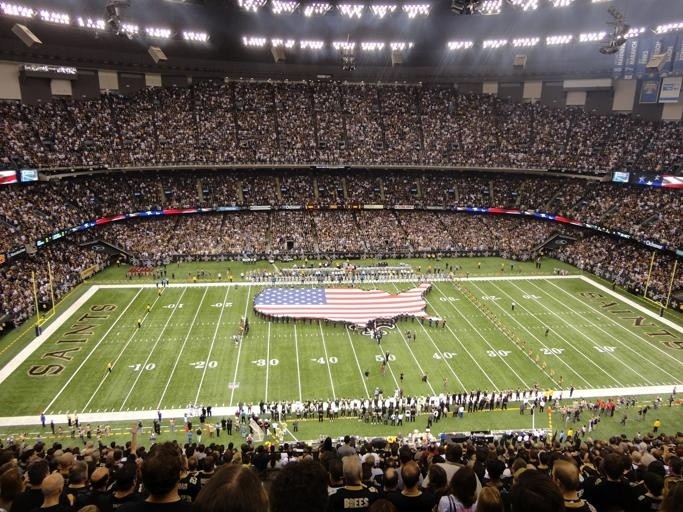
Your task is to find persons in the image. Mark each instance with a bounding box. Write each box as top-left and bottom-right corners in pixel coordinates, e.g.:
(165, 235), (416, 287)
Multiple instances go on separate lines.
(1, 79), (682, 512)
